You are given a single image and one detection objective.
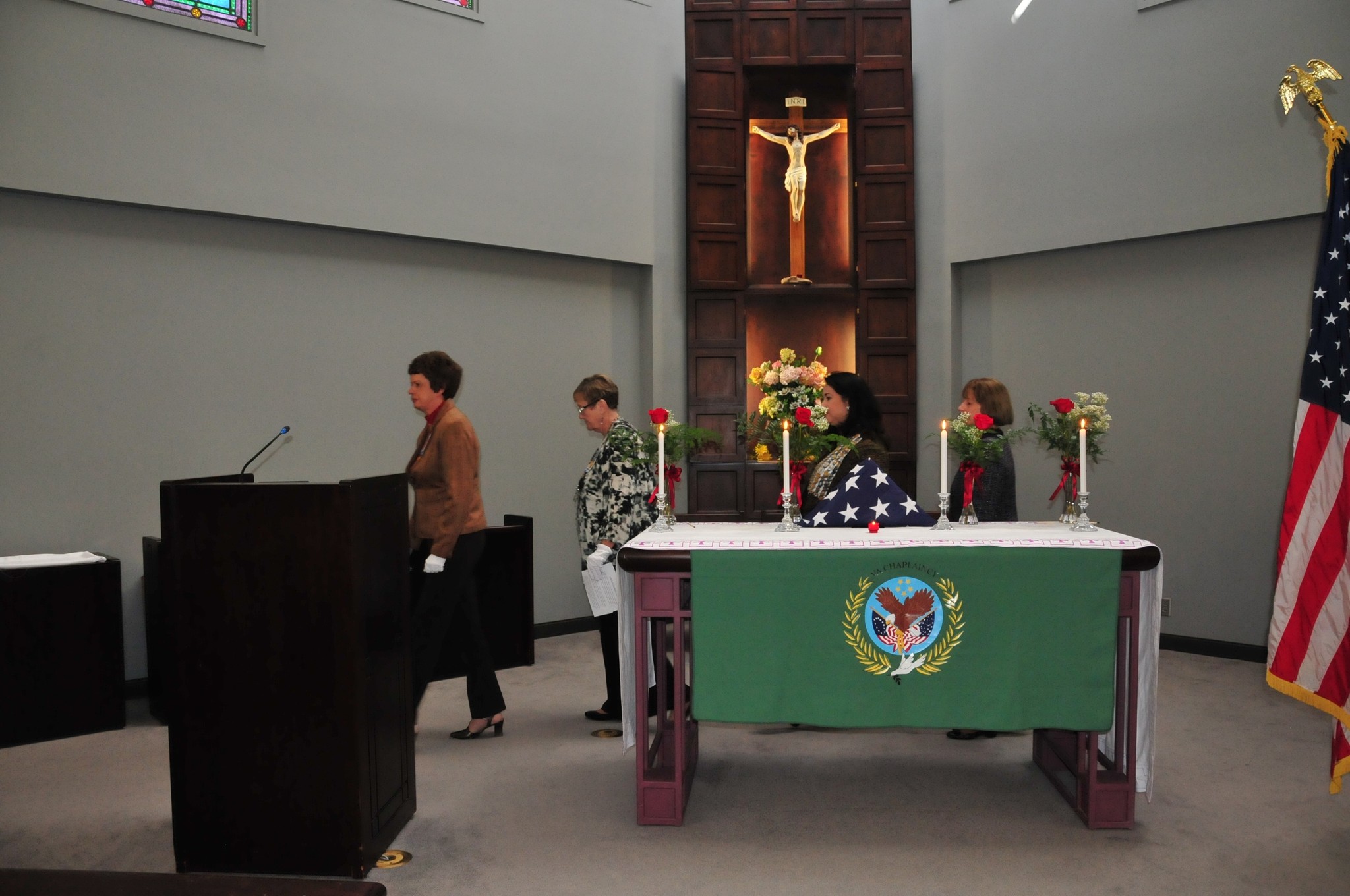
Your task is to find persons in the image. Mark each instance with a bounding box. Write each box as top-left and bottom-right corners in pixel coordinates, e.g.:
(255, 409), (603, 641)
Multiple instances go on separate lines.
(752, 122), (841, 222)
(947, 378), (1019, 522)
(405, 349), (506, 741)
(573, 375), (690, 722)
(799, 371), (892, 517)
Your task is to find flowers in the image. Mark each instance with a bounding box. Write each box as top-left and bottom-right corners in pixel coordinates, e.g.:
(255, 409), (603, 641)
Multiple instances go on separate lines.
(743, 347), (861, 456)
(928, 411), (1025, 462)
(1028, 391), (1114, 460)
(608, 407), (724, 465)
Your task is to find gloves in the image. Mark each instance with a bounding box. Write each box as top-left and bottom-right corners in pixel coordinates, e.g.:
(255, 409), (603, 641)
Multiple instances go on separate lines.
(422, 553), (448, 573)
(586, 543), (612, 581)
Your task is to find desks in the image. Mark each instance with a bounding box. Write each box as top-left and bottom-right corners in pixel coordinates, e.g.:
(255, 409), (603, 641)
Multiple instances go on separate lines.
(0, 549), (124, 754)
(615, 523), (1163, 828)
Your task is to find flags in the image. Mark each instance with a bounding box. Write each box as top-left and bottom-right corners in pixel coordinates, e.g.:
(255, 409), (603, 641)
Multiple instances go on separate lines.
(1264, 139), (1350, 795)
(799, 457), (939, 529)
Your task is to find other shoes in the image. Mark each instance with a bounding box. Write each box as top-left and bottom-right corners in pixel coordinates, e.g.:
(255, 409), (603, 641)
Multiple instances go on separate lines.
(947, 728), (995, 738)
(585, 708), (616, 720)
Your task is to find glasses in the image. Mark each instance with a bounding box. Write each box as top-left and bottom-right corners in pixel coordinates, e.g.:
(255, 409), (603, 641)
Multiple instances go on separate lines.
(577, 401), (598, 413)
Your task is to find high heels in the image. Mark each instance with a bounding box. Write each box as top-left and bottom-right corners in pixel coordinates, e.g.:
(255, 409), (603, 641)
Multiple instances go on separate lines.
(451, 713), (505, 740)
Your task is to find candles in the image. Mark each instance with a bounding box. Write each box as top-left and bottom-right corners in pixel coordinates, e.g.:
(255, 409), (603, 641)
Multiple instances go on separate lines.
(783, 421), (790, 492)
(941, 420), (947, 492)
(657, 425), (665, 493)
(1080, 418), (1086, 492)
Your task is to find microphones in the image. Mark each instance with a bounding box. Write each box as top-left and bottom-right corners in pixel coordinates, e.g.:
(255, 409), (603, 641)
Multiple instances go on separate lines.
(239, 425), (290, 482)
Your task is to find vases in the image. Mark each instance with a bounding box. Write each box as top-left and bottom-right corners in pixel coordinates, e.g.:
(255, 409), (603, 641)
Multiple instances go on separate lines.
(782, 463), (804, 528)
(659, 470), (677, 525)
(1049, 460), (1079, 523)
(959, 460), (979, 524)
